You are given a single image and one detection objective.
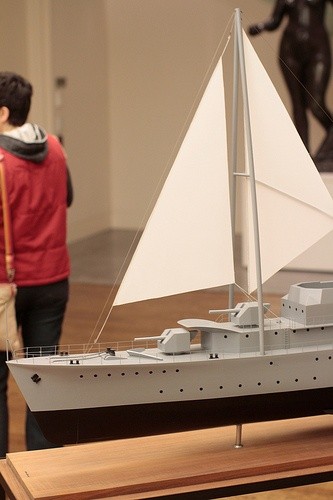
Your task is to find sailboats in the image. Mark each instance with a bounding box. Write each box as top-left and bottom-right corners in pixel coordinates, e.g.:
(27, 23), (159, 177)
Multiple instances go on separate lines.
(2, 8), (333, 451)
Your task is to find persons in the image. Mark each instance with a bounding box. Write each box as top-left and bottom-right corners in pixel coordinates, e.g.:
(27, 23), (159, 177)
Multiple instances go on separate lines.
(0, 72), (73, 458)
(248, 0), (333, 163)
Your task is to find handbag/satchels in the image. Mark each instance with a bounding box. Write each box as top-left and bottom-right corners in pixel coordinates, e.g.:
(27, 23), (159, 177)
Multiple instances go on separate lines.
(1, 282), (23, 351)
